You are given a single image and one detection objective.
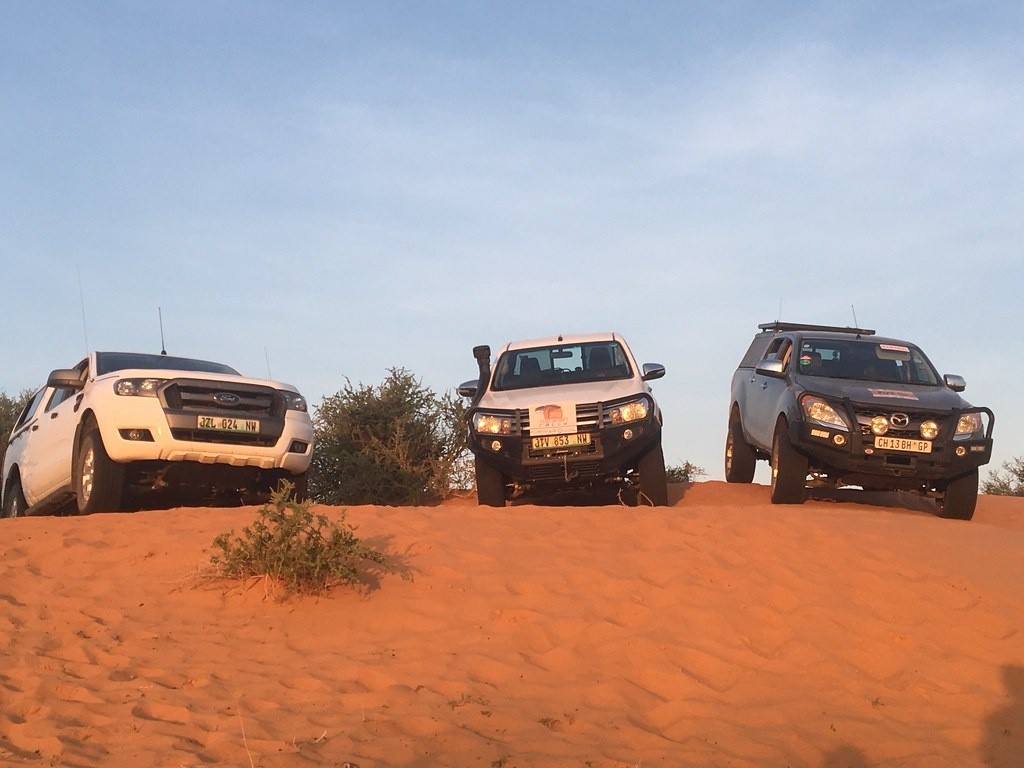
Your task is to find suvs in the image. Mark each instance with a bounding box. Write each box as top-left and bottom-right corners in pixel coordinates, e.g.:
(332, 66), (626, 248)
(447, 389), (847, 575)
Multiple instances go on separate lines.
(456, 332), (668, 506)
(723, 321), (996, 520)
(3, 309), (314, 523)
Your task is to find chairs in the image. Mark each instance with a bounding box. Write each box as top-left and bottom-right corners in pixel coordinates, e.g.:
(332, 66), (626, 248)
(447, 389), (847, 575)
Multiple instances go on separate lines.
(801, 351), (822, 371)
(520, 358), (541, 376)
(589, 347), (613, 370)
